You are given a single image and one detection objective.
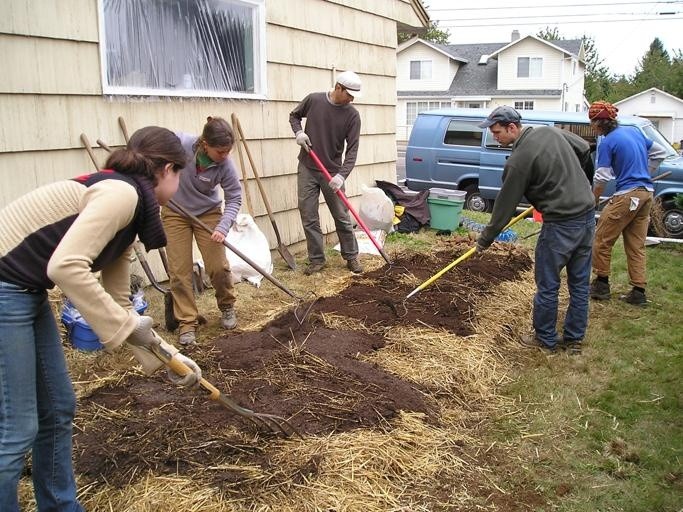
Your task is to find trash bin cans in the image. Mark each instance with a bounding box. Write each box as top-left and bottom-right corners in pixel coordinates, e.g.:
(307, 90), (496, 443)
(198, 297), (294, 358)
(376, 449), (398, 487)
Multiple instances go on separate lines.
(238, 117), (301, 270)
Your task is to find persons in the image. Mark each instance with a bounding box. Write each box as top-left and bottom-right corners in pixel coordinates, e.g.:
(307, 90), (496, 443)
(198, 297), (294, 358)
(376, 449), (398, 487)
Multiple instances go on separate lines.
(588, 101), (668, 305)
(157, 115), (242, 346)
(475, 105), (595, 357)
(1, 127), (200, 512)
(288, 71), (364, 274)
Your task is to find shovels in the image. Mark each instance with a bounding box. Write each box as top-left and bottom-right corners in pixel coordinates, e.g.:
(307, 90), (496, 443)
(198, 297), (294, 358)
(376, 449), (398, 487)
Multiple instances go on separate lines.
(80, 131), (178, 331)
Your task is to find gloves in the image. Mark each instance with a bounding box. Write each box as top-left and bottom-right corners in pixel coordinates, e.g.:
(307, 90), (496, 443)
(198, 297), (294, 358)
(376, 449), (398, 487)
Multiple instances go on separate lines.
(164, 343), (203, 389)
(126, 315), (161, 347)
(295, 129), (312, 153)
(327, 173), (346, 194)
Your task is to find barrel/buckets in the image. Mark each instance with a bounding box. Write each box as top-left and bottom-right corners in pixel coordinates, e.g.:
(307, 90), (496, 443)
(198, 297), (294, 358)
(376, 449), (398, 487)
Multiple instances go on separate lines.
(532, 209), (543, 223)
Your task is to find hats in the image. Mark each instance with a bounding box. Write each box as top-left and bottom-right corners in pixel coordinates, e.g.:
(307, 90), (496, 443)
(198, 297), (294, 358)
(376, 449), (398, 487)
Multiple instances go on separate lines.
(588, 100), (619, 120)
(478, 105), (522, 129)
(336, 70), (362, 97)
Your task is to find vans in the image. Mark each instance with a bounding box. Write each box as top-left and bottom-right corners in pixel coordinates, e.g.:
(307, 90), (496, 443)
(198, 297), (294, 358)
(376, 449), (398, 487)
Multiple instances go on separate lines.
(405, 110), (683, 240)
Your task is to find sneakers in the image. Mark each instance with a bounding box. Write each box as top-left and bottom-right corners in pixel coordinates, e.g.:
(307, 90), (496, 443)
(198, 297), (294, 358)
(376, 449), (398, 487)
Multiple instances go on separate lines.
(618, 286), (647, 307)
(303, 258), (326, 275)
(557, 335), (582, 355)
(179, 324), (196, 346)
(519, 334), (556, 354)
(220, 306), (238, 330)
(346, 257), (362, 274)
(588, 279), (611, 300)
